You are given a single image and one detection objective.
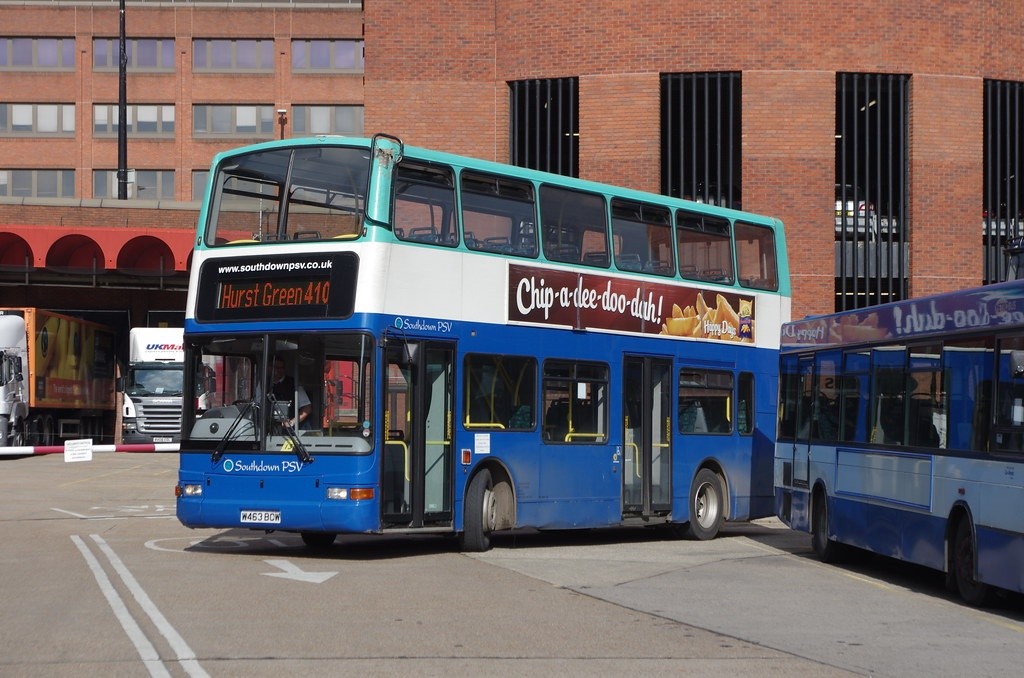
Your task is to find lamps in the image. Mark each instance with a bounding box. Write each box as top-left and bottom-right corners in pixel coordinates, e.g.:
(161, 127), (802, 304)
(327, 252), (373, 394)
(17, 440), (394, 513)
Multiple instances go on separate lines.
(277, 109), (287, 120)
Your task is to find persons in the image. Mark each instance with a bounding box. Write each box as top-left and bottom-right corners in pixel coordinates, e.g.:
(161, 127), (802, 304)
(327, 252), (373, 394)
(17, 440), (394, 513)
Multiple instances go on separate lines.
(254, 356), (312, 437)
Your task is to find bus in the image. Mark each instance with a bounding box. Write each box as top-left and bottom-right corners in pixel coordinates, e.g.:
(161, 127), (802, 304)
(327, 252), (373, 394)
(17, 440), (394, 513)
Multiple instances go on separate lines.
(175, 132), (792, 557)
(774, 280), (1024, 606)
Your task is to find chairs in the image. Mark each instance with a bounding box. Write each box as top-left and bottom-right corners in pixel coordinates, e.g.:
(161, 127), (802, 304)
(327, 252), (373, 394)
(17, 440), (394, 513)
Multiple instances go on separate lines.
(504, 396), (752, 442)
(795, 390), (941, 447)
(268, 227), (753, 288)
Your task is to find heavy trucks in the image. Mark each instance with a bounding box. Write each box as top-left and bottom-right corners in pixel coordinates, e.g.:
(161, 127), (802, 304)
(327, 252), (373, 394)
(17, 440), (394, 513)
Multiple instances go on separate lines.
(117, 327), (217, 444)
(0, 307), (121, 447)
